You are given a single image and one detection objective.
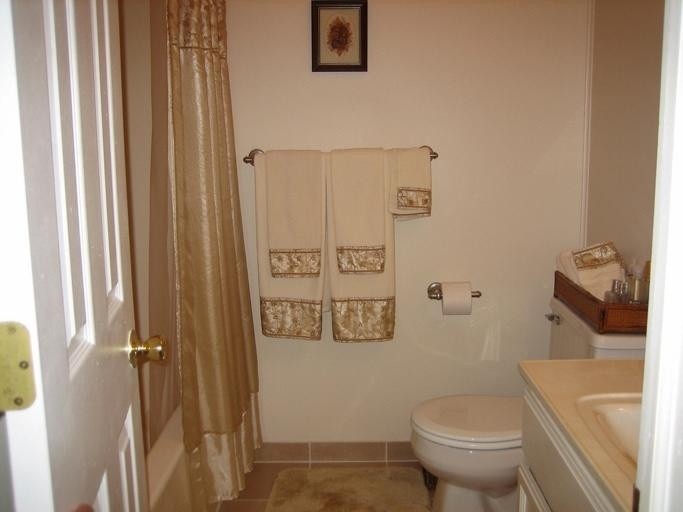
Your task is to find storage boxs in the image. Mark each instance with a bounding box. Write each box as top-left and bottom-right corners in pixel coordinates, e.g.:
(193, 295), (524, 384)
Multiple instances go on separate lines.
(555, 272), (648, 335)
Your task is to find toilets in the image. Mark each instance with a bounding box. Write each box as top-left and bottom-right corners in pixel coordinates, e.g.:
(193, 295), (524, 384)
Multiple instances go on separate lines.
(409, 394), (522, 511)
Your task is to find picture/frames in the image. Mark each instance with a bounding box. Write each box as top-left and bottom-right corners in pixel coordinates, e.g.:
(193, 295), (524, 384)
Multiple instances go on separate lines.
(313, 0), (368, 73)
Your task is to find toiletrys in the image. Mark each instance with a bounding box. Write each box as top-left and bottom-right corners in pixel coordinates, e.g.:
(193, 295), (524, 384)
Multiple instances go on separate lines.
(604, 259), (651, 305)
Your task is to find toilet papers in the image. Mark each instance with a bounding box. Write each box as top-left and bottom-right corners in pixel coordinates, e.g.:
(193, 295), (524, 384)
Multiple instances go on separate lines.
(440, 282), (473, 316)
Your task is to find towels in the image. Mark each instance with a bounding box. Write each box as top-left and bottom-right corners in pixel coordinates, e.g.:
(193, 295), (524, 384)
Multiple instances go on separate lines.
(254, 146), (434, 343)
(557, 241), (627, 298)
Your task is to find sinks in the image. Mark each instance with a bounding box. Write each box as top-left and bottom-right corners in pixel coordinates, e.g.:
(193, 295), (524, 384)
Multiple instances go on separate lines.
(575, 393), (641, 478)
